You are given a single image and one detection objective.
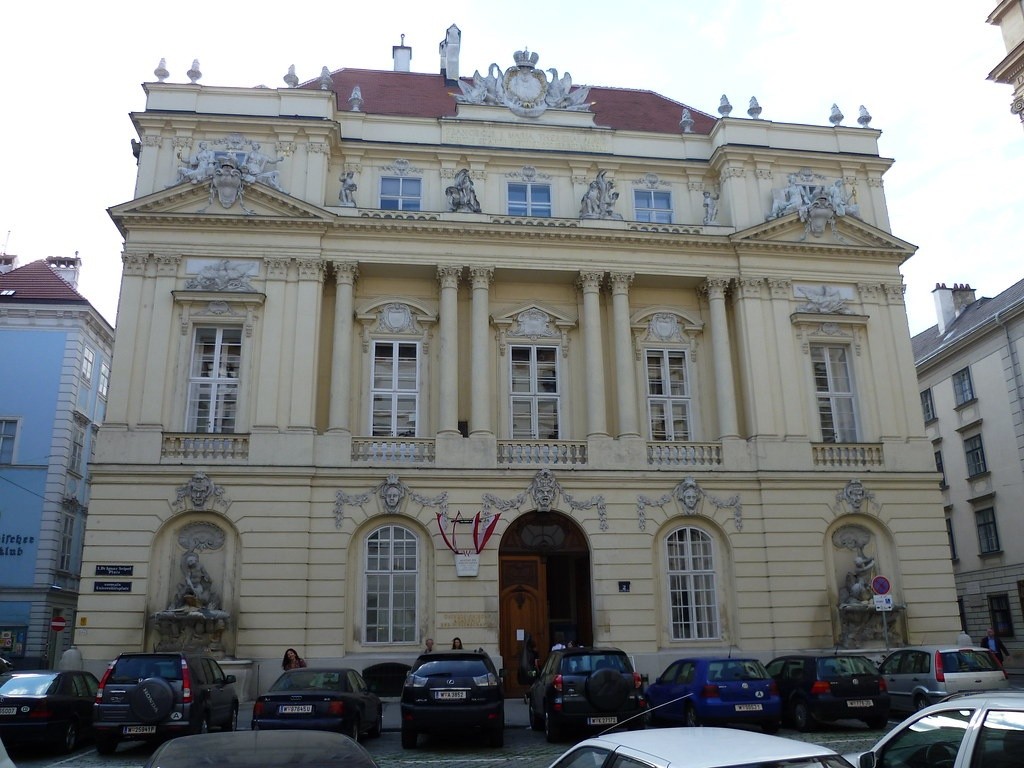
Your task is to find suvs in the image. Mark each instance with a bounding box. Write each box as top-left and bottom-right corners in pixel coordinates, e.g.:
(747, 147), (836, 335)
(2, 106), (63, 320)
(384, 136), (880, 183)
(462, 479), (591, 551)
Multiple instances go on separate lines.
(399, 647), (510, 750)
(91, 648), (240, 754)
(525, 646), (647, 742)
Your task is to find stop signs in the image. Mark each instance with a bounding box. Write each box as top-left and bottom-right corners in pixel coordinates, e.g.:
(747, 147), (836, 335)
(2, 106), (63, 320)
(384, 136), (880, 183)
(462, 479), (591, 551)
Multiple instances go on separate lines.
(51, 617), (67, 632)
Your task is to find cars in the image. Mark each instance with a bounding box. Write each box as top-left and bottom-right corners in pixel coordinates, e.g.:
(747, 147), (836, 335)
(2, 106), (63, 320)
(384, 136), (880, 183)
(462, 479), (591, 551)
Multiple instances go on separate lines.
(546, 728), (859, 768)
(252, 666), (385, 745)
(805, 690), (1024, 768)
(766, 652), (893, 730)
(0, 657), (16, 673)
(644, 656), (783, 733)
(0, 669), (100, 754)
(880, 644), (1008, 714)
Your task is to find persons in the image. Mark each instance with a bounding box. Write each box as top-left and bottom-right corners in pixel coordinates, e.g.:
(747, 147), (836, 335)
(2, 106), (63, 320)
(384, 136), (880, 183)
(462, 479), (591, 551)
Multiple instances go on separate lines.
(380, 474), (404, 514)
(183, 557), (213, 602)
(452, 637), (463, 650)
(981, 628), (1009, 668)
(189, 471), (212, 507)
(422, 639), (435, 653)
(518, 640), (539, 706)
(531, 467), (557, 511)
(551, 640), (566, 652)
(163, 141), (214, 189)
(584, 182), (600, 214)
(239, 143), (291, 195)
(566, 641), (574, 648)
(767, 174), (860, 221)
(677, 476), (701, 508)
(337, 171), (358, 207)
(701, 191), (720, 224)
(844, 481), (866, 510)
(851, 557), (877, 607)
(456, 177), (477, 209)
(282, 648), (307, 671)
